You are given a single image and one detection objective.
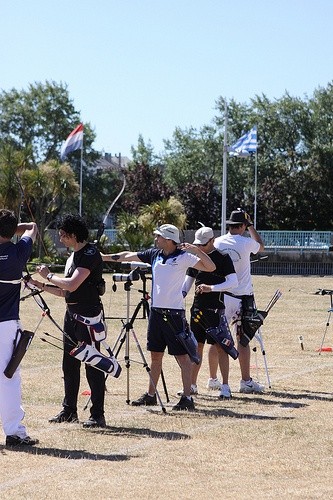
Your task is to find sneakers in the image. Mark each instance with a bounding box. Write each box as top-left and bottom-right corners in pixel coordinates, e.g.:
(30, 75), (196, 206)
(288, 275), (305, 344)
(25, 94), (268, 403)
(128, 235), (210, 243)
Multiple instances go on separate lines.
(6, 436), (39, 445)
(207, 377), (222, 388)
(83, 414), (106, 428)
(219, 384), (232, 399)
(131, 392), (157, 406)
(177, 386), (198, 395)
(49, 409), (79, 423)
(172, 395), (195, 411)
(239, 377), (265, 393)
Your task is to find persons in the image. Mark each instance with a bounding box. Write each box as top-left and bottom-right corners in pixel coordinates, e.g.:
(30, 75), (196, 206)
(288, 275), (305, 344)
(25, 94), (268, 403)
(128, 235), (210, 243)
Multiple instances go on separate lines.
(0, 209), (39, 446)
(100, 224), (216, 410)
(208, 211), (265, 393)
(177, 227), (238, 399)
(21, 213), (107, 428)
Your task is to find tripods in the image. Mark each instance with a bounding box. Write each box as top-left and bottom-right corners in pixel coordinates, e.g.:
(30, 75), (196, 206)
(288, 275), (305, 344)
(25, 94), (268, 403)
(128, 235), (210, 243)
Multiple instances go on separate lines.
(84, 283), (168, 414)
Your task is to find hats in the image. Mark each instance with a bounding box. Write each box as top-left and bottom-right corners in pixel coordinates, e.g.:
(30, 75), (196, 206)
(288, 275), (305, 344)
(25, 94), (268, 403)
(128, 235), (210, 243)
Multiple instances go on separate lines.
(226, 211), (253, 226)
(153, 224), (181, 243)
(192, 227), (214, 244)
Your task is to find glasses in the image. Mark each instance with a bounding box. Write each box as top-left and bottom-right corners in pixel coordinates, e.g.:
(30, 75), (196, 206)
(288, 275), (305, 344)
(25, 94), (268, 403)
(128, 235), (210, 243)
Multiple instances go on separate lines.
(197, 237), (214, 247)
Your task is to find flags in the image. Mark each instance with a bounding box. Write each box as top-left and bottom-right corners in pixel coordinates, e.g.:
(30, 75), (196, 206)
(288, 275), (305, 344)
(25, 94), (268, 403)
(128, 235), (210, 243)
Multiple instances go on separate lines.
(229, 126), (257, 156)
(62, 124), (83, 160)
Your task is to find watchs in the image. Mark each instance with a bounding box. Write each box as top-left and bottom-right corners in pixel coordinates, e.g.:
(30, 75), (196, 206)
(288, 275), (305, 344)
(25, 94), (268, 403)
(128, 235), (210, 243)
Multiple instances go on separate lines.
(47, 273), (53, 280)
